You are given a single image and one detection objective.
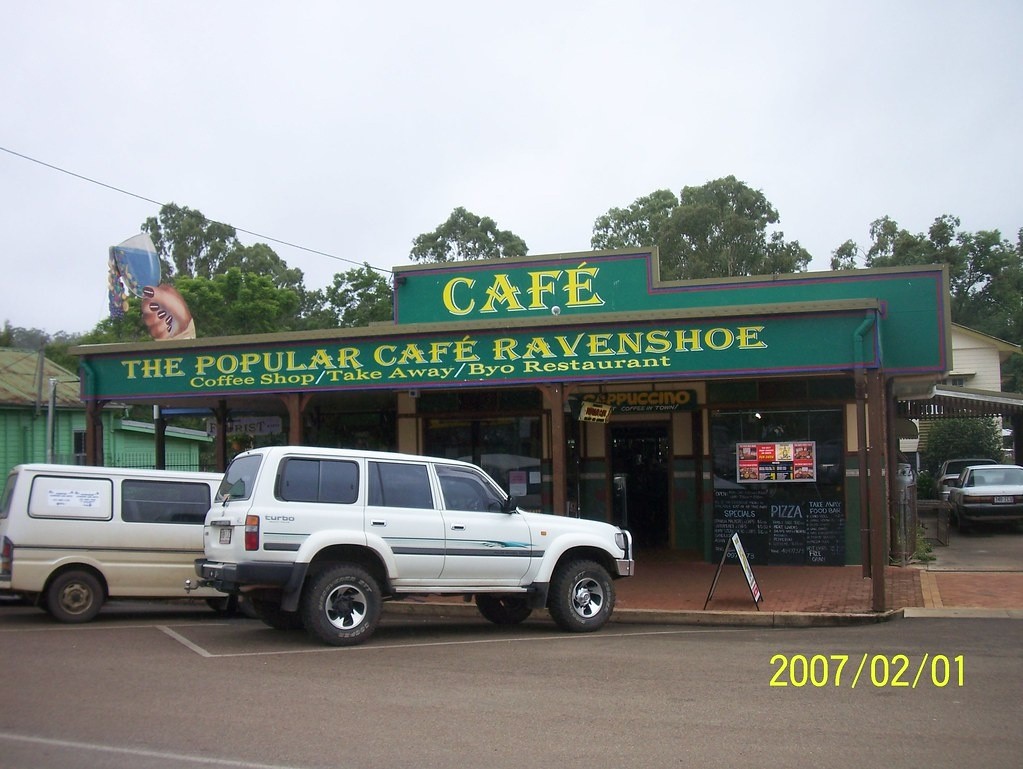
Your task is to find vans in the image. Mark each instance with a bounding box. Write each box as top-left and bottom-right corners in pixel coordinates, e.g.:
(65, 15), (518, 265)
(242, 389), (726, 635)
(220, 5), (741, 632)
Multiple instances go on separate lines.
(0, 463), (250, 623)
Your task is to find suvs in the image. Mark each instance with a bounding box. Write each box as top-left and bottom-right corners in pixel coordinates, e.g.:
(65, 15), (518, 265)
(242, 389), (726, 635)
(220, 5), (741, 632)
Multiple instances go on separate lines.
(184, 445), (635, 646)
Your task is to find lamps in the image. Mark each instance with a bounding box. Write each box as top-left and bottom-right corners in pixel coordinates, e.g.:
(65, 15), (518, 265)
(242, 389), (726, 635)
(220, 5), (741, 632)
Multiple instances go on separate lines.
(397, 276), (407, 285)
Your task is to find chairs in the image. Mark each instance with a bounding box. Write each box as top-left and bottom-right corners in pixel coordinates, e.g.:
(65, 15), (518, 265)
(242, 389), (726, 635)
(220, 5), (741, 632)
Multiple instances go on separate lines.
(971, 475), (985, 485)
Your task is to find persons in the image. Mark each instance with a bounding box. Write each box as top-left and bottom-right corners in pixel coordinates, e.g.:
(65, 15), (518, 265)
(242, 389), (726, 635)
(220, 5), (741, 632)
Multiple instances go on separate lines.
(230, 438), (245, 464)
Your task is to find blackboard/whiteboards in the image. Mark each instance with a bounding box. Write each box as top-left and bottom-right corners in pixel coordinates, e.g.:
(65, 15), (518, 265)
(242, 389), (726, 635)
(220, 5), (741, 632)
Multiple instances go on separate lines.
(713, 487), (844, 568)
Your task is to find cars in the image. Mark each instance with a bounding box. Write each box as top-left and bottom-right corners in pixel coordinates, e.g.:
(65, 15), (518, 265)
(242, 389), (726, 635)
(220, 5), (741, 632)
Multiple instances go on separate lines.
(947, 465), (1023, 532)
(937, 459), (998, 505)
